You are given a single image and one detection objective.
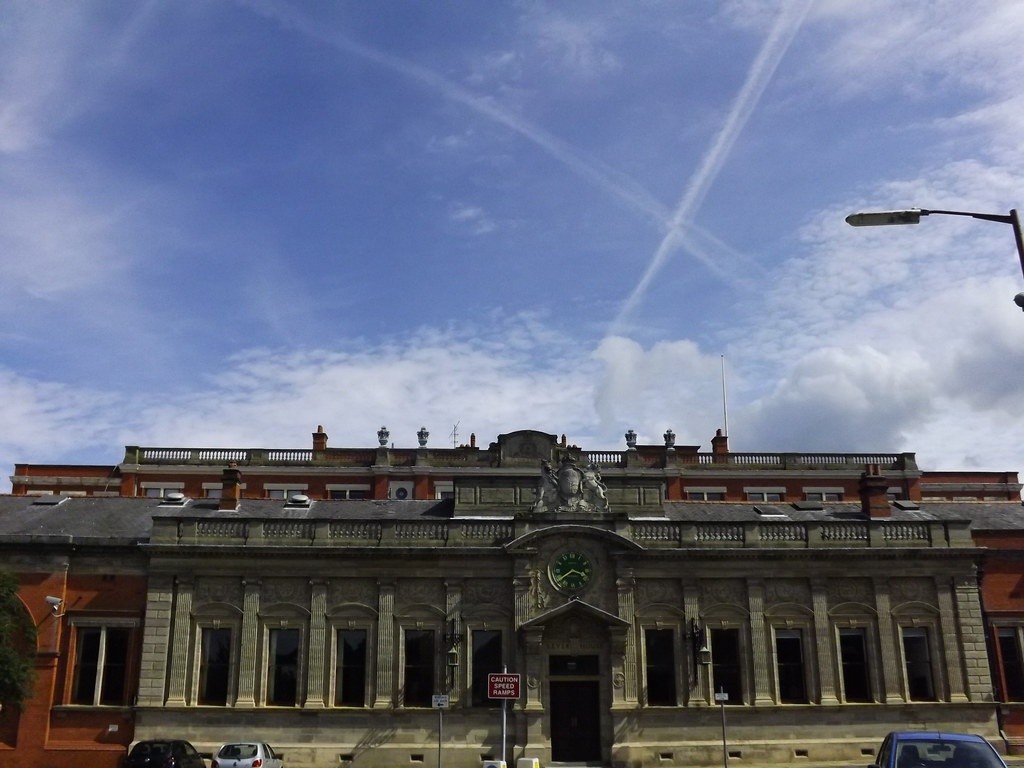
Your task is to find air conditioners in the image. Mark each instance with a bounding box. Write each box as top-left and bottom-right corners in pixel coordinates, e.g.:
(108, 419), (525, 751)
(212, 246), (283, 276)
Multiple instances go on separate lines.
(388, 480), (415, 501)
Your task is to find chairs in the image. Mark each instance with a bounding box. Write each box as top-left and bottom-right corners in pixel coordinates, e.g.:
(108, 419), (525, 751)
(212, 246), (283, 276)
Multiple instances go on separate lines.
(945, 746), (978, 768)
(231, 747), (241, 756)
(149, 747), (161, 756)
(897, 745), (921, 768)
(251, 749), (256, 756)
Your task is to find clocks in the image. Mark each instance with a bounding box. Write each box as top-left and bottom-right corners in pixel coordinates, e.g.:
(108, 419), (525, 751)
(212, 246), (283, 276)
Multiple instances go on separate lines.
(547, 544), (598, 597)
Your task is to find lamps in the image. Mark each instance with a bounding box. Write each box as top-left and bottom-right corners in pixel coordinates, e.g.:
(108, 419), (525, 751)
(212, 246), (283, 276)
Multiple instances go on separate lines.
(698, 646), (712, 666)
(447, 647), (459, 666)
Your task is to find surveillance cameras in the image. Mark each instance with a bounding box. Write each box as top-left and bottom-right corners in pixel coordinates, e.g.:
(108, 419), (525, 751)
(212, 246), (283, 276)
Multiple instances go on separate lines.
(46, 596), (63, 605)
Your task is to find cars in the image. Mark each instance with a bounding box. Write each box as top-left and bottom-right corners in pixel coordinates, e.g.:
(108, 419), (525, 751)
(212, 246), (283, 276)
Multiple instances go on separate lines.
(210, 742), (284, 768)
(125, 738), (207, 768)
(868, 730), (1008, 768)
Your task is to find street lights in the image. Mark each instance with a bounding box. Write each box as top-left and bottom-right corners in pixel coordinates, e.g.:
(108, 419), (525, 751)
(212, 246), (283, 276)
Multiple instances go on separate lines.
(845, 206), (1024, 309)
